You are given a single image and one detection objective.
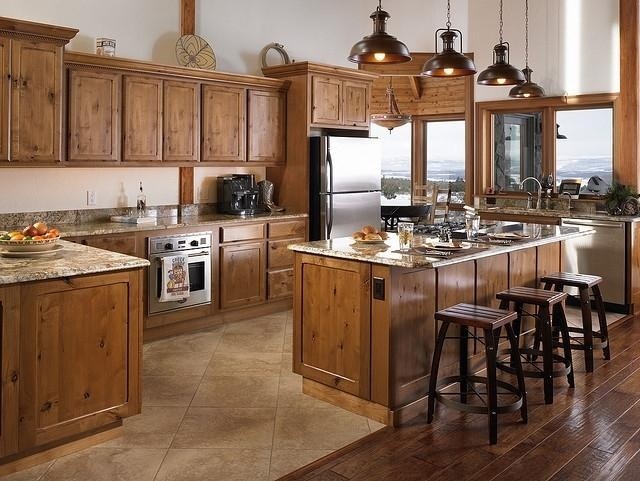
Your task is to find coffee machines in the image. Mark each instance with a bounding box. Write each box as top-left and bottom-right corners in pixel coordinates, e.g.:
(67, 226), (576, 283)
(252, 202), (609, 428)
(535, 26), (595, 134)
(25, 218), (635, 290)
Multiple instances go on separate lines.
(217, 173), (266, 216)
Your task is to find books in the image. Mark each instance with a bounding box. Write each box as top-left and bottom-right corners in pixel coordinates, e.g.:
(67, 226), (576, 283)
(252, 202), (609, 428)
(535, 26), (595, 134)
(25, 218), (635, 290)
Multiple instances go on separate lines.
(111, 216), (154, 224)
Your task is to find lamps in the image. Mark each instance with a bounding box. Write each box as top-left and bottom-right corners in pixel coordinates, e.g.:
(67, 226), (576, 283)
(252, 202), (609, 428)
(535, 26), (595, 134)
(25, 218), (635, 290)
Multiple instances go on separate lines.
(421, 0), (477, 77)
(347, 0), (411, 65)
(477, 0), (526, 87)
(509, 0), (546, 98)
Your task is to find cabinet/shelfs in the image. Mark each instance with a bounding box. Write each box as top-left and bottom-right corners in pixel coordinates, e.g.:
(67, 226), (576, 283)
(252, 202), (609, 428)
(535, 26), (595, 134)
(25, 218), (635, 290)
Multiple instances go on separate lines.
(200, 69), (291, 166)
(217, 222), (267, 325)
(63, 51), (119, 167)
(0, 17), (79, 168)
(120, 58), (200, 167)
(267, 214), (309, 315)
(261, 61), (379, 129)
(1, 261), (151, 478)
(289, 224), (598, 427)
(60, 228), (142, 348)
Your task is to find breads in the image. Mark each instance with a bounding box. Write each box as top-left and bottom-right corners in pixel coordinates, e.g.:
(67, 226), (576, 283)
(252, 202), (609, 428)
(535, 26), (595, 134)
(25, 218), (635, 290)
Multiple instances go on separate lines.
(364, 233), (382, 240)
(352, 231), (366, 240)
(378, 231), (387, 239)
(363, 225), (374, 234)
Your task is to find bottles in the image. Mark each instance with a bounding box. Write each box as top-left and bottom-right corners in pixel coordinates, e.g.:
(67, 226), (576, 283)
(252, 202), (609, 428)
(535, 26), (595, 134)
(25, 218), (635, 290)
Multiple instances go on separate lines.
(137, 181), (147, 218)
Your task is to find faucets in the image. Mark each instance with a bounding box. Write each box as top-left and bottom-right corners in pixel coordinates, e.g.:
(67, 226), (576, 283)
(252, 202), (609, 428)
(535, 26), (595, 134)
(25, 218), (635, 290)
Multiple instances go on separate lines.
(518, 175), (543, 208)
(561, 191), (575, 210)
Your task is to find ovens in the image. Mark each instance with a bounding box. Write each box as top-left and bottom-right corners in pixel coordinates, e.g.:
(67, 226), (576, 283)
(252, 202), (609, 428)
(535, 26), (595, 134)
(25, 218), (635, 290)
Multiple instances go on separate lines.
(148, 231), (212, 317)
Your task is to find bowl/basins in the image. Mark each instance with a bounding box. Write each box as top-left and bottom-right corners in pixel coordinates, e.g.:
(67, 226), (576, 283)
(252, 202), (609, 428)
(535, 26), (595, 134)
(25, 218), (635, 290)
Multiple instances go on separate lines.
(0, 236), (60, 252)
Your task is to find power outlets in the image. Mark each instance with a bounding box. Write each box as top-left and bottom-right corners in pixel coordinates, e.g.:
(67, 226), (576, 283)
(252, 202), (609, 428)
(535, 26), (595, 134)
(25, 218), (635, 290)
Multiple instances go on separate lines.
(88, 191), (96, 204)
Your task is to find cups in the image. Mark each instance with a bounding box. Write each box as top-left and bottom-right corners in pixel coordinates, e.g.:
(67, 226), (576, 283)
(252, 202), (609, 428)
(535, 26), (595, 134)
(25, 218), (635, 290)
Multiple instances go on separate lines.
(397, 222), (414, 253)
(465, 215), (481, 241)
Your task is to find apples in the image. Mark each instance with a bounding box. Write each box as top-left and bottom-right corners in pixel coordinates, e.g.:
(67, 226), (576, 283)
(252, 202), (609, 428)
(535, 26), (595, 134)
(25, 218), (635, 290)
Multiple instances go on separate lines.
(24, 226), (38, 236)
(42, 233), (48, 239)
(34, 221), (47, 236)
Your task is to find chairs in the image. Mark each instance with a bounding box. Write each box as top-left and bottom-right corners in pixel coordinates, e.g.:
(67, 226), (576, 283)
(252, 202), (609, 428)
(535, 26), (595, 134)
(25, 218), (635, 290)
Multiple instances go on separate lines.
(411, 182), (452, 229)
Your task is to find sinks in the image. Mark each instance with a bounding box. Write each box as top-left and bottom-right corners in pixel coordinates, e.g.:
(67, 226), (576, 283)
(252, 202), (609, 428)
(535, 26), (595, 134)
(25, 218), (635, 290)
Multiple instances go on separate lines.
(501, 207), (541, 212)
(542, 211), (578, 214)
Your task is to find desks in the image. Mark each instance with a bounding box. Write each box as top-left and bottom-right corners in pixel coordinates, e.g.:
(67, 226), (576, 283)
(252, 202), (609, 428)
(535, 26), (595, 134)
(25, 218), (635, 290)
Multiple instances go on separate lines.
(381, 204), (432, 231)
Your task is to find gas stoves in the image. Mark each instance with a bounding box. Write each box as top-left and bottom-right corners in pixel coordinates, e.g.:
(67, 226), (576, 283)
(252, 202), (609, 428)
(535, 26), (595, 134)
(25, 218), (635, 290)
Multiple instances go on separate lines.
(415, 221), (494, 238)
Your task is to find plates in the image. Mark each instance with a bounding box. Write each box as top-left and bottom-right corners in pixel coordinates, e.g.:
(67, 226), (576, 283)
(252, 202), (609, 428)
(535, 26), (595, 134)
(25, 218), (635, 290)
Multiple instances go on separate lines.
(355, 239), (386, 245)
(0, 244), (65, 258)
(175, 34), (217, 70)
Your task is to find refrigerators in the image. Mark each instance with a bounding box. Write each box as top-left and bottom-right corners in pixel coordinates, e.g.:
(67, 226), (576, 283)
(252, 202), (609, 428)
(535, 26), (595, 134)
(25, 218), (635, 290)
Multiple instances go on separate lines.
(309, 135), (381, 241)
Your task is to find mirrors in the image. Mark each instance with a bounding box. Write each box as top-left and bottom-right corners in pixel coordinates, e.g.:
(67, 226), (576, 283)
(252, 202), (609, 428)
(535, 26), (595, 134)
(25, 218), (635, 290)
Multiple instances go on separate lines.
(475, 93), (619, 201)
(412, 114), (474, 210)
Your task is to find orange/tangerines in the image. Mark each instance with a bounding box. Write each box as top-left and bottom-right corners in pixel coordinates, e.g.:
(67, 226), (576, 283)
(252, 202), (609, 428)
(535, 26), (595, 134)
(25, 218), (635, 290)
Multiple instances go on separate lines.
(24, 234), (32, 241)
(0, 233), (10, 240)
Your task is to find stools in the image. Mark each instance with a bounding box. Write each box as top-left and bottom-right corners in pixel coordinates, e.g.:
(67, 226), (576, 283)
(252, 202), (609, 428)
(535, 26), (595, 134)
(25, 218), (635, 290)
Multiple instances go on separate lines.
(427, 272), (611, 445)
